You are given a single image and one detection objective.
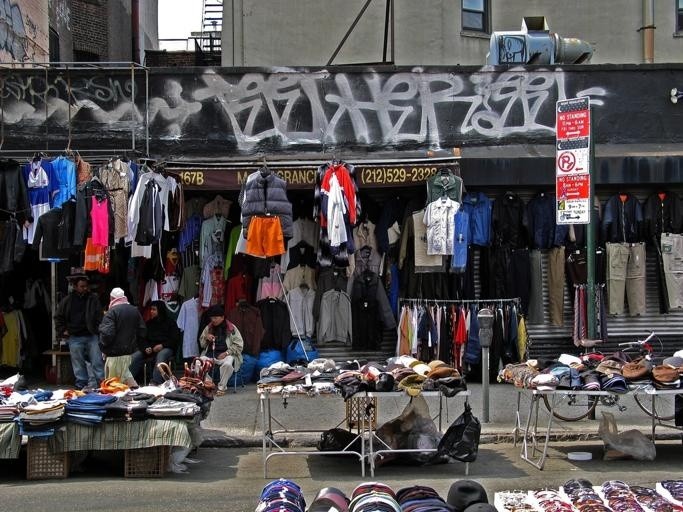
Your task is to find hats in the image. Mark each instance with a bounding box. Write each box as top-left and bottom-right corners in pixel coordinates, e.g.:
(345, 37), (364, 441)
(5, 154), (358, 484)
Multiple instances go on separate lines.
(259, 477), (496, 512)
(111, 287), (124, 298)
(207, 305), (224, 316)
(505, 350), (683, 393)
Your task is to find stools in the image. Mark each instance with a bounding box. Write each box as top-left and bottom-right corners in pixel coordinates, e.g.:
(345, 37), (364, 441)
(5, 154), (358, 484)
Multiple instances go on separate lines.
(142, 357), (174, 386)
(211, 365), (246, 393)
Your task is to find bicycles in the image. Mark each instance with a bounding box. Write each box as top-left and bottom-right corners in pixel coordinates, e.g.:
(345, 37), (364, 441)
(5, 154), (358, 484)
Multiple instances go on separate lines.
(543, 328), (677, 420)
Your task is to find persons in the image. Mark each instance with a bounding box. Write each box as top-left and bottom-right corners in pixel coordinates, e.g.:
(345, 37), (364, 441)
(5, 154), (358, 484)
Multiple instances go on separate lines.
(130, 300), (179, 386)
(53, 277), (105, 389)
(97, 287), (141, 387)
(197, 306), (244, 396)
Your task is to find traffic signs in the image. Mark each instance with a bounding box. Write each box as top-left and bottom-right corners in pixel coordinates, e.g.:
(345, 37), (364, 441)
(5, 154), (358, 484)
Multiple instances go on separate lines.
(556, 136), (589, 150)
(554, 198), (591, 225)
(556, 149), (589, 176)
(555, 110), (590, 139)
(555, 96), (590, 113)
(555, 174), (590, 199)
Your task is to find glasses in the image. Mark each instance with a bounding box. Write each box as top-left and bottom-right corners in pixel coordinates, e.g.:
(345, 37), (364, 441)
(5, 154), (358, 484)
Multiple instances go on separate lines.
(535, 479), (682, 511)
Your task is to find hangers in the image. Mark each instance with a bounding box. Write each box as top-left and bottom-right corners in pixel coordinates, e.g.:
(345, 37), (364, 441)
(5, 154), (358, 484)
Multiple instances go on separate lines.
(0, 152), (183, 215)
(426, 160), (682, 212)
(398, 296), (518, 317)
(257, 148), (375, 298)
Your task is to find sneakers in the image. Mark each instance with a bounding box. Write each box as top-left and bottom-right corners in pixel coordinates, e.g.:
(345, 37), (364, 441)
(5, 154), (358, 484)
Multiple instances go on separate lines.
(217, 390), (225, 396)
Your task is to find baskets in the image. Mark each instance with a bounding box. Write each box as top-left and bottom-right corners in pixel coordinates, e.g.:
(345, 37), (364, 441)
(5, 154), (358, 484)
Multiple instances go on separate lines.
(346, 398), (377, 430)
(124, 447), (168, 478)
(27, 436), (68, 480)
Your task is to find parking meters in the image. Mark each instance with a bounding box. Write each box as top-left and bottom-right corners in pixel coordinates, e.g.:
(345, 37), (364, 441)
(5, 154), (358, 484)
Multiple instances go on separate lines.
(474, 307), (493, 425)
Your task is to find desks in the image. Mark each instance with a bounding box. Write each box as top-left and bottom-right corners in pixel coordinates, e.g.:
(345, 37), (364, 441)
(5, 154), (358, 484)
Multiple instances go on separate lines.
(513, 385), (683, 471)
(259, 390), (473, 477)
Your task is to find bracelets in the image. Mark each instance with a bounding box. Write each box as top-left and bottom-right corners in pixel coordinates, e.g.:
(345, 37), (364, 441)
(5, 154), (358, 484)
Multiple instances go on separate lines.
(226, 351), (230, 355)
